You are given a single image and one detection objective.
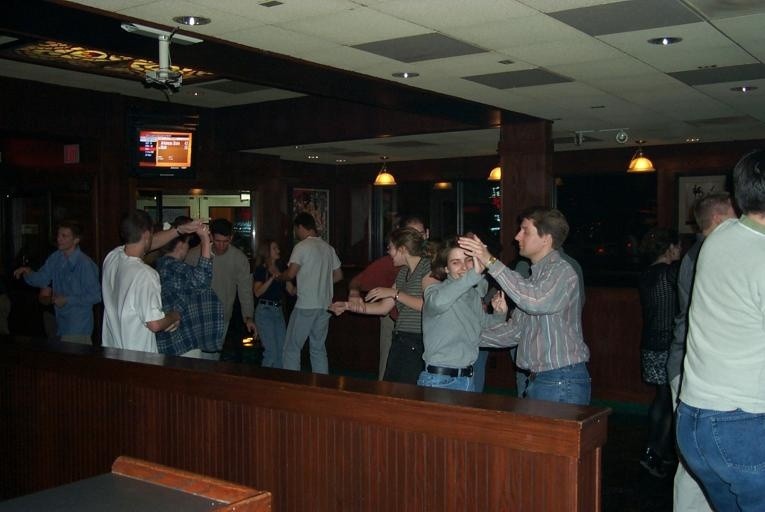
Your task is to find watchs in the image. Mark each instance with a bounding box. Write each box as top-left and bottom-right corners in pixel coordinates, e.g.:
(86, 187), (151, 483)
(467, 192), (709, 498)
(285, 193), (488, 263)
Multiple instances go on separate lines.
(485, 256), (497, 267)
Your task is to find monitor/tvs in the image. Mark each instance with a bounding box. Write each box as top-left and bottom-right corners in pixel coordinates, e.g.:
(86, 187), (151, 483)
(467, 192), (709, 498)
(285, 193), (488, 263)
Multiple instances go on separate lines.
(125, 113), (199, 179)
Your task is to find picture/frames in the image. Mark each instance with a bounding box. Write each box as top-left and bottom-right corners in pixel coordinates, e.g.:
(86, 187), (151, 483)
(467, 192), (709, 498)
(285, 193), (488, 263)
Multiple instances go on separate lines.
(671, 165), (730, 242)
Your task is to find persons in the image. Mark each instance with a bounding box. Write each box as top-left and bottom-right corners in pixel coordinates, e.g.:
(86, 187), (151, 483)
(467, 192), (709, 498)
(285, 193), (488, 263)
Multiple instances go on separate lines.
(274, 212), (343, 374)
(39, 279), (57, 337)
(636, 148), (765, 512)
(102, 207), (205, 353)
(458, 206), (590, 404)
(202, 218), (257, 360)
(14, 221), (101, 345)
(174, 216), (193, 229)
(328, 213), (508, 392)
(152, 222), (224, 359)
(514, 261), (530, 279)
(14, 233), (48, 337)
(252, 238), (297, 368)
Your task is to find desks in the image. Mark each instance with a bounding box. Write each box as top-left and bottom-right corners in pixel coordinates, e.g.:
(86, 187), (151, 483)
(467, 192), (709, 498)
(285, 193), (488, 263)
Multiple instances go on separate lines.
(2, 452), (277, 512)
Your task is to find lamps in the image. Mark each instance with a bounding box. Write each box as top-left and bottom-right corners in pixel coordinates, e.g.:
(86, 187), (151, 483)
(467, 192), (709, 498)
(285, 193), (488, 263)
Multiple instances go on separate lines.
(373, 153), (403, 191)
(624, 137), (657, 178)
(573, 127), (630, 147)
(482, 140), (503, 183)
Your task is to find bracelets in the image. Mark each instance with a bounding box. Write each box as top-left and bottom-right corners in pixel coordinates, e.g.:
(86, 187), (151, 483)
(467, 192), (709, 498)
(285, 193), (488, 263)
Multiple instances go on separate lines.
(175, 226), (180, 234)
(394, 290), (400, 299)
(50, 296), (52, 304)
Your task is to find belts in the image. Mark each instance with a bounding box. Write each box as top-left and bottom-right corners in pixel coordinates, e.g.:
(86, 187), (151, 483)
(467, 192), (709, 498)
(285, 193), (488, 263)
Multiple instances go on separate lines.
(428, 366), (473, 377)
(261, 301), (281, 307)
